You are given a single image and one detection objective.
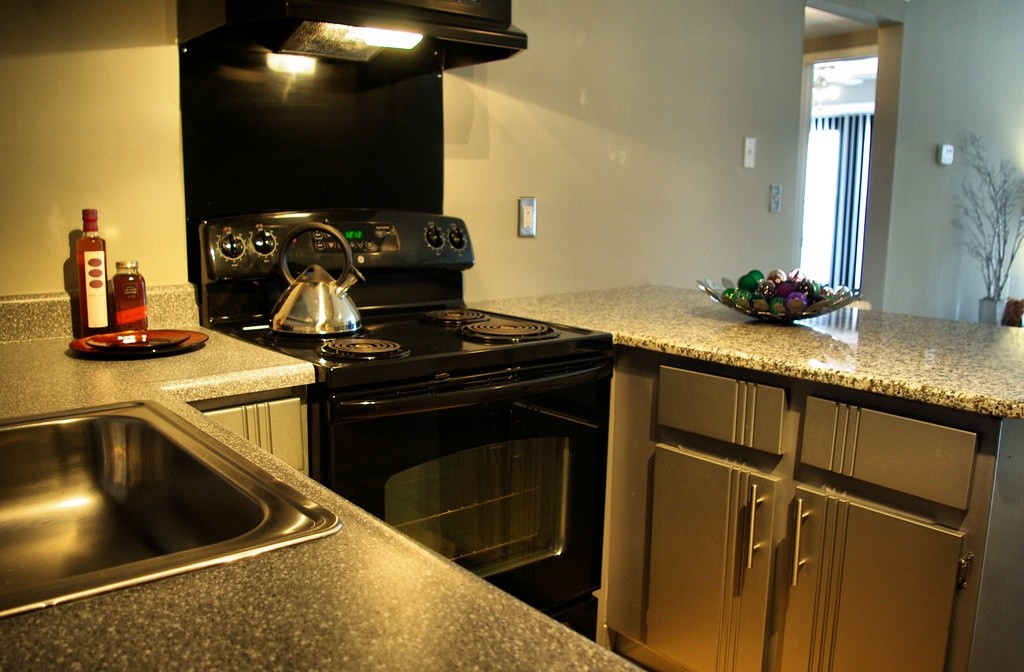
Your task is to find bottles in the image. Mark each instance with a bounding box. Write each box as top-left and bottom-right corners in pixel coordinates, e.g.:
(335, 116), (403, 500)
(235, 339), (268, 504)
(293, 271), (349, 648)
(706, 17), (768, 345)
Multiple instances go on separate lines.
(76, 209), (109, 338)
(112, 261), (148, 332)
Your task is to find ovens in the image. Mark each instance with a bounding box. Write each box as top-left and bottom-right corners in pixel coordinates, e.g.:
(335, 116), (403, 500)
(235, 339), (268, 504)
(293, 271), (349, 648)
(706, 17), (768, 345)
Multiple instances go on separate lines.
(308, 363), (614, 623)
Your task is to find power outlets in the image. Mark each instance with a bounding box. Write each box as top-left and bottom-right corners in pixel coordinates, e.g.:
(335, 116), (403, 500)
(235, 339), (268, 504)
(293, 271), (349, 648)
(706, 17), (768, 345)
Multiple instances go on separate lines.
(519, 198), (537, 236)
(743, 137), (756, 167)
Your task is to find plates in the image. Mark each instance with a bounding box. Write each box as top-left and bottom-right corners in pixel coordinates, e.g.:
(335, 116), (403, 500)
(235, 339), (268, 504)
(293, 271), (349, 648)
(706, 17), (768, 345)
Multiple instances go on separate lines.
(85, 330), (190, 351)
(69, 331), (209, 358)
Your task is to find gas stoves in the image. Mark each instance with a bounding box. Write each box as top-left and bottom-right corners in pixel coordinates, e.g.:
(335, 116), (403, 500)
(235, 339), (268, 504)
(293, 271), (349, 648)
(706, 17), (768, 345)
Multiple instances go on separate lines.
(201, 210), (614, 390)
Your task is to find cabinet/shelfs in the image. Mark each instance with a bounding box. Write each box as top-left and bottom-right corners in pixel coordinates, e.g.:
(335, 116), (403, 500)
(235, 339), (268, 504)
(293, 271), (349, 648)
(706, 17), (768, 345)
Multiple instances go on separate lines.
(186, 390), (309, 478)
(604, 340), (1024, 672)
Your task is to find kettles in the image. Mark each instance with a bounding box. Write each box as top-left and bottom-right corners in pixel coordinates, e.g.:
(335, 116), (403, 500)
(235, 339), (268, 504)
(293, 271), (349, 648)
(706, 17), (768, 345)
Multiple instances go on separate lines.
(269, 222), (366, 339)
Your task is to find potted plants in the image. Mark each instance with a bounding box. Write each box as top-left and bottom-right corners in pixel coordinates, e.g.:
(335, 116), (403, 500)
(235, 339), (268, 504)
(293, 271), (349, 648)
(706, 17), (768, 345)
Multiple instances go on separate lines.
(951, 132), (1024, 323)
